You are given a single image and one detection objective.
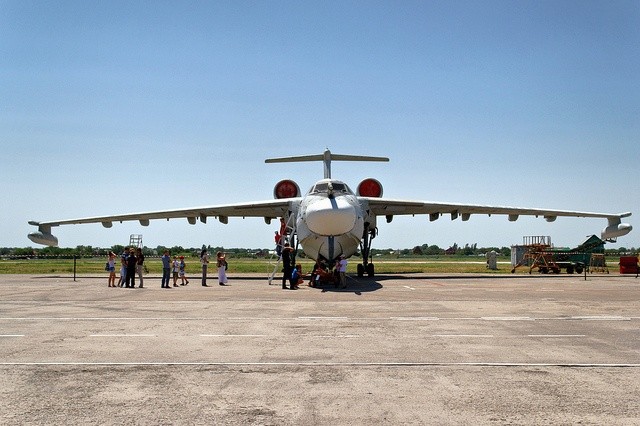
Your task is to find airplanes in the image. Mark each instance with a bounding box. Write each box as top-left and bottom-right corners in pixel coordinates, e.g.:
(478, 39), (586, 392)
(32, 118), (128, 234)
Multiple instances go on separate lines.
(25, 147), (634, 293)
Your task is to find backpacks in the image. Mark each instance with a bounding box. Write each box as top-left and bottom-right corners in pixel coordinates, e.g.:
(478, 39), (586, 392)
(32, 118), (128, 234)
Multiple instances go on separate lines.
(104, 262), (111, 271)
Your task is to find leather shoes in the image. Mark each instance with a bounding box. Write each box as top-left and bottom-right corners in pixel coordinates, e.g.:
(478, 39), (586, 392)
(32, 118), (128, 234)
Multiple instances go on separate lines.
(162, 286), (165, 287)
(167, 286), (171, 288)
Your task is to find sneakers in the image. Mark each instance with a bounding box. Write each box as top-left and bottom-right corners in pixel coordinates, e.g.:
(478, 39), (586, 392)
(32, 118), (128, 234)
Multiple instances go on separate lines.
(186, 281), (189, 284)
(180, 283), (184, 285)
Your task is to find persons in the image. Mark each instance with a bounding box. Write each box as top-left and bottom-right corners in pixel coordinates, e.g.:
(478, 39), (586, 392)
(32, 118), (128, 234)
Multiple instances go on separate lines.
(336, 258), (341, 287)
(126, 248), (137, 288)
(161, 250), (172, 288)
(120, 256), (128, 287)
(179, 256), (189, 286)
(280, 217), (287, 237)
(275, 231), (282, 261)
(108, 251), (116, 287)
(282, 243), (297, 290)
(118, 247), (129, 286)
(216, 252), (228, 286)
(292, 263), (309, 288)
(340, 256), (347, 289)
(200, 250), (209, 286)
(309, 259), (326, 285)
(172, 255), (179, 287)
(136, 248), (144, 288)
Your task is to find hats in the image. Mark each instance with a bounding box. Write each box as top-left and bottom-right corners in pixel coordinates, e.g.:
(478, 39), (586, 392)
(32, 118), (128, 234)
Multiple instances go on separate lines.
(340, 255), (347, 258)
(178, 255), (185, 259)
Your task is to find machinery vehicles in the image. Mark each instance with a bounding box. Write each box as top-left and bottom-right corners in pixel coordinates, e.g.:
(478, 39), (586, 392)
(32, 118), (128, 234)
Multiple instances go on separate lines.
(511, 244), (585, 274)
